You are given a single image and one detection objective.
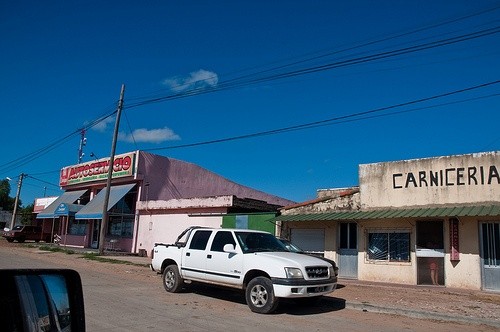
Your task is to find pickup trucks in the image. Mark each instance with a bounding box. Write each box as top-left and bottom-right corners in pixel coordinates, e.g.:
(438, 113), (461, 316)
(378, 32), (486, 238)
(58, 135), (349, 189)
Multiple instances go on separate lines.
(2, 224), (55, 243)
(150, 225), (339, 315)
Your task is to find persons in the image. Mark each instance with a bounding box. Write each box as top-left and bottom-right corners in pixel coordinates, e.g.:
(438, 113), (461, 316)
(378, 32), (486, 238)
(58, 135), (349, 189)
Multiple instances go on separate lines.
(426, 242), (443, 285)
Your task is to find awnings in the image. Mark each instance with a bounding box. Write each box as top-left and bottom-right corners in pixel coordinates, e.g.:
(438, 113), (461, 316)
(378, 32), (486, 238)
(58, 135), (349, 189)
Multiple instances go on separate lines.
(265, 203), (500, 228)
(37, 183), (138, 219)
